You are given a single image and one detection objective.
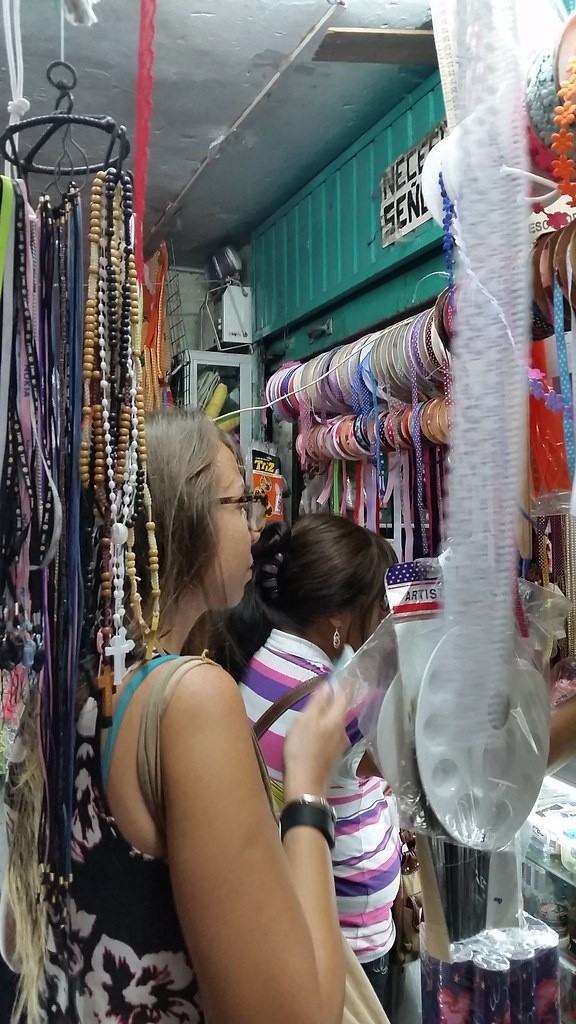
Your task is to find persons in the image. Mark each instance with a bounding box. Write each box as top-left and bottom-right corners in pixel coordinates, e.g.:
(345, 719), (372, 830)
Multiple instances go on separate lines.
(217, 513), (576, 1000)
(0, 407), (348, 1024)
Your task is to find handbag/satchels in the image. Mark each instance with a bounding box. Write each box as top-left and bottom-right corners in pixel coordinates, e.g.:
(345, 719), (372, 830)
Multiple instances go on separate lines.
(395, 847), (424, 965)
(137, 658), (390, 1024)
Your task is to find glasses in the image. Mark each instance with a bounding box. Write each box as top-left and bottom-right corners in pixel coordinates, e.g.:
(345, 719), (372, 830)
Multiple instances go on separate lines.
(214, 493), (272, 533)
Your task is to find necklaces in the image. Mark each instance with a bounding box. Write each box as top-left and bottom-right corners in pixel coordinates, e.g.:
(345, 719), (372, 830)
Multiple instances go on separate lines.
(0, 168), (160, 681)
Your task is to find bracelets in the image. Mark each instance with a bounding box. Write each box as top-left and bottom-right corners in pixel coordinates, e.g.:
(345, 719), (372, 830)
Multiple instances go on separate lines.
(278, 794), (337, 850)
